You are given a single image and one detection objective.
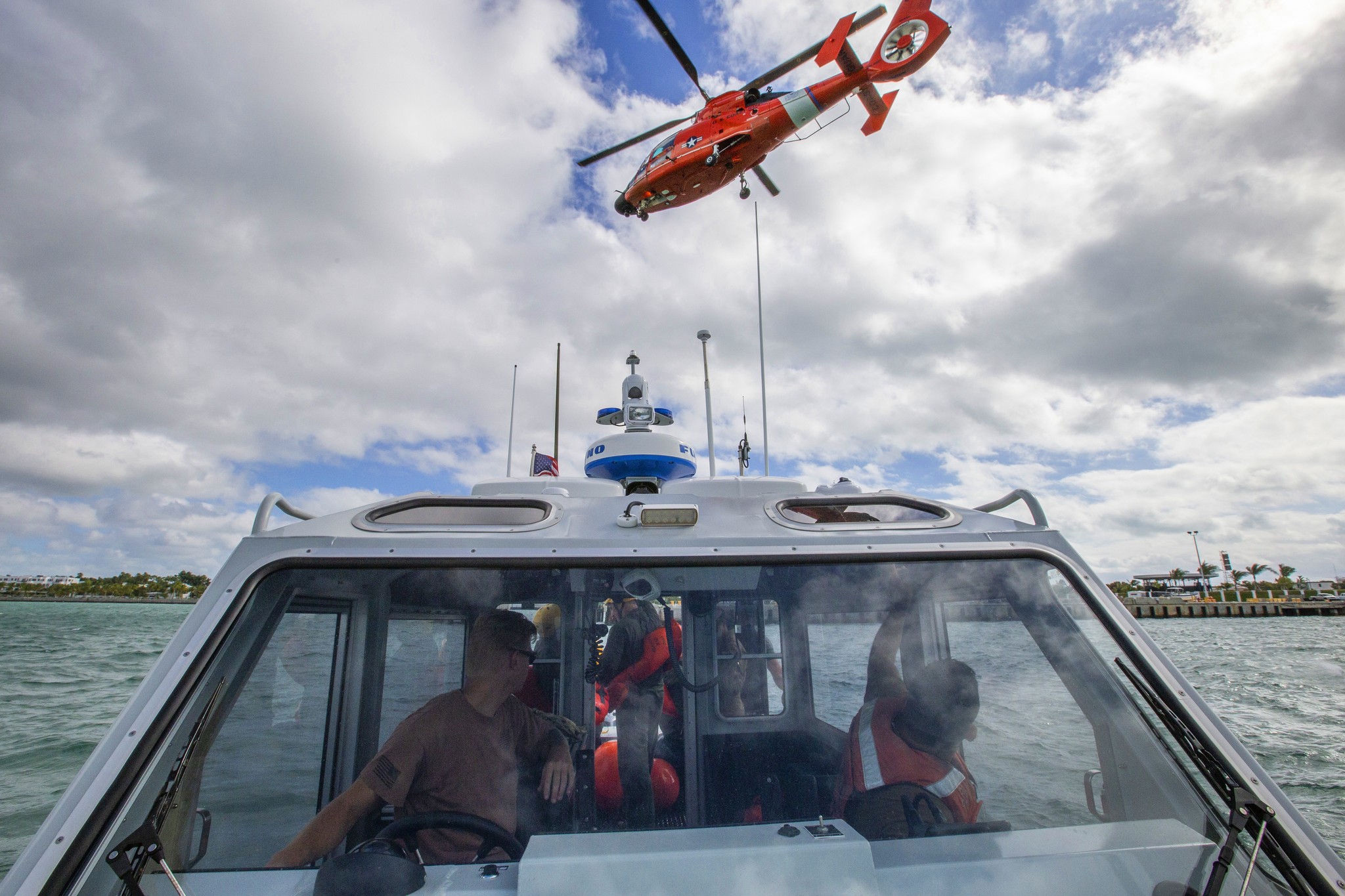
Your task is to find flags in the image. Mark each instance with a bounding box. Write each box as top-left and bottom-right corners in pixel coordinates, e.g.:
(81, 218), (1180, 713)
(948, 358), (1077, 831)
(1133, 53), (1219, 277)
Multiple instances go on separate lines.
(533, 452), (560, 478)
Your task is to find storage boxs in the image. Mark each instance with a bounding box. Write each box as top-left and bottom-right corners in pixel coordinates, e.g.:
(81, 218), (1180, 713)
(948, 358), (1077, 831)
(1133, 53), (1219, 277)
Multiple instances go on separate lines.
(1204, 596), (1300, 602)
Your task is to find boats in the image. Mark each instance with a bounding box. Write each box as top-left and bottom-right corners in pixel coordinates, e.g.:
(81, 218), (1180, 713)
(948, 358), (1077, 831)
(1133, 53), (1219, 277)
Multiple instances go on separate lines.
(0, 330), (1343, 896)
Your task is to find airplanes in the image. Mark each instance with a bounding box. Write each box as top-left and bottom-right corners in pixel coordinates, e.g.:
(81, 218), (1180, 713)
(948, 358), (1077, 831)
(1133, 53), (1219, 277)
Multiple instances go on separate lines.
(576, 0), (943, 224)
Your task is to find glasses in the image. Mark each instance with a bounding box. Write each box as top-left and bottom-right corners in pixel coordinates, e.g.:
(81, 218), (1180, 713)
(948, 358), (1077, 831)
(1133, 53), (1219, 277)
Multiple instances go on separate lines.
(507, 647), (537, 665)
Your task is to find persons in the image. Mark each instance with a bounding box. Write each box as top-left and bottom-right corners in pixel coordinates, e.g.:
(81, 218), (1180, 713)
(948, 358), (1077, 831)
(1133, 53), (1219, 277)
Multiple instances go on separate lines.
(830, 577), (984, 826)
(595, 595), (670, 836)
(266, 608), (578, 867)
(517, 604), (784, 720)
